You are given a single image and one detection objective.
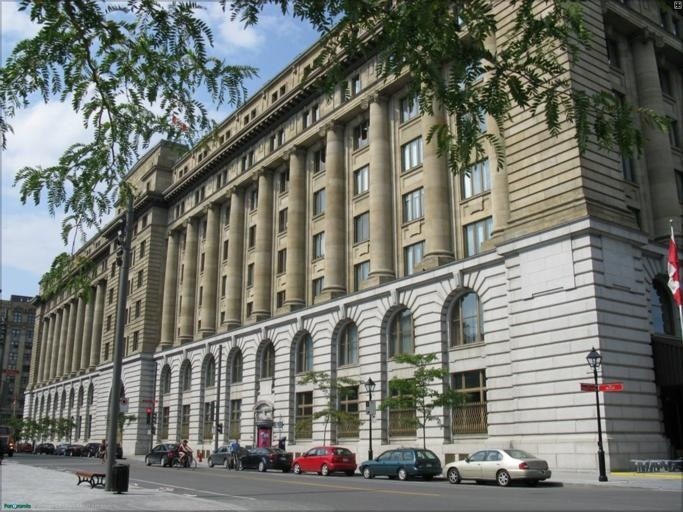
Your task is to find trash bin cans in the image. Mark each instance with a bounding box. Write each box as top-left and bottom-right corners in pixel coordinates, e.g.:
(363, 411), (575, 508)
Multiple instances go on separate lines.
(109, 463), (130, 493)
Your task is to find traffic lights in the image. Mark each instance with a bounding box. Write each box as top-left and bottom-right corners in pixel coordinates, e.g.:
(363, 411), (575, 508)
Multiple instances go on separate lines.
(147, 407), (151, 425)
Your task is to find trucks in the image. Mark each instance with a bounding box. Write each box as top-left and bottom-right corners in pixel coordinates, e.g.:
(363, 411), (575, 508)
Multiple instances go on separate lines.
(0, 425), (14, 457)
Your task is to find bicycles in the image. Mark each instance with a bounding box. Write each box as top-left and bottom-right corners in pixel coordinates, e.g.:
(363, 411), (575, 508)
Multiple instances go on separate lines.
(172, 451), (197, 471)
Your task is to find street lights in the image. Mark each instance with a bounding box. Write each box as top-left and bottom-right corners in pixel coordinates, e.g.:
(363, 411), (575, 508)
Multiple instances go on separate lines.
(364, 377), (376, 461)
(587, 347), (609, 481)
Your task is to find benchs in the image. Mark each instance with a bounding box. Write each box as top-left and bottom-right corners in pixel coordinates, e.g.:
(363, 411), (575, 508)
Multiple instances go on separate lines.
(71, 468), (106, 490)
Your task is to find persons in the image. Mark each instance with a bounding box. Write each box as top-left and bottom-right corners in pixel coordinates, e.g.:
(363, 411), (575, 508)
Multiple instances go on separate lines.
(175, 439), (193, 462)
(229, 438), (240, 455)
(97, 440), (108, 457)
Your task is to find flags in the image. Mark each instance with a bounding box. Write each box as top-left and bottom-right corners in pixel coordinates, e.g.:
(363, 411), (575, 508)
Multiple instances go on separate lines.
(664, 226), (683, 306)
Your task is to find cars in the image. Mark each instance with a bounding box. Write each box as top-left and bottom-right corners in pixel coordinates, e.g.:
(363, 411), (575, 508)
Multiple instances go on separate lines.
(18, 442), (124, 457)
(208, 445), (293, 473)
(290, 446), (357, 475)
(359, 447), (443, 481)
(145, 442), (180, 467)
(442, 449), (551, 486)
